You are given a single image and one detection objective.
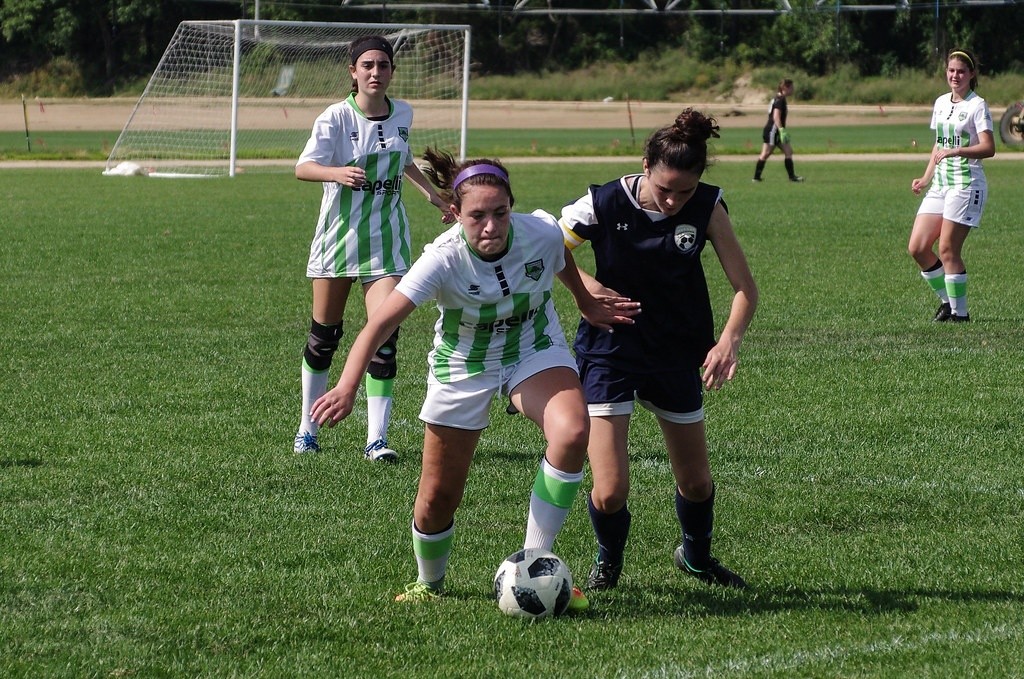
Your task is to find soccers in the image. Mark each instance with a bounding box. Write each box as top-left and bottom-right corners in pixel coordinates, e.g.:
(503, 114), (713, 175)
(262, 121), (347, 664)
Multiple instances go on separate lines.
(492, 547), (575, 619)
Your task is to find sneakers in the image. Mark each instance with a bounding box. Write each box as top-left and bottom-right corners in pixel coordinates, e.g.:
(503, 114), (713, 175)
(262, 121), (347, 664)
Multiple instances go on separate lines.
(675, 544), (746, 590)
(364, 439), (399, 462)
(945, 312), (970, 323)
(294, 430), (319, 454)
(566, 586), (589, 611)
(396, 574), (447, 602)
(584, 554), (623, 589)
(933, 302), (951, 322)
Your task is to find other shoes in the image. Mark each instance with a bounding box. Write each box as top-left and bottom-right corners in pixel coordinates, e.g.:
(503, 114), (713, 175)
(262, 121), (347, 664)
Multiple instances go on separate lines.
(752, 177), (764, 182)
(788, 176), (804, 181)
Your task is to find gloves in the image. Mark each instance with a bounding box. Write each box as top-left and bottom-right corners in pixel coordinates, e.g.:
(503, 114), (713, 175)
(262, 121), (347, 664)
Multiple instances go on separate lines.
(779, 127), (790, 145)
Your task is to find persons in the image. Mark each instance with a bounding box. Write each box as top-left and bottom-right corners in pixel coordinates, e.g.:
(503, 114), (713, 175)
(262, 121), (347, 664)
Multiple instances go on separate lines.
(752, 79), (804, 182)
(908, 47), (996, 323)
(559, 109), (759, 590)
(294, 35), (456, 463)
(308, 144), (643, 612)
